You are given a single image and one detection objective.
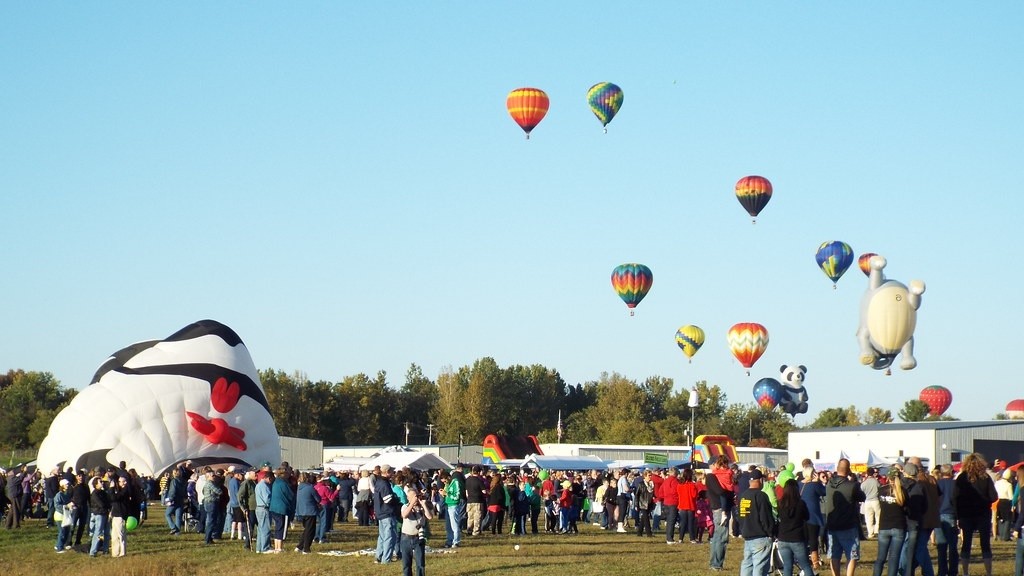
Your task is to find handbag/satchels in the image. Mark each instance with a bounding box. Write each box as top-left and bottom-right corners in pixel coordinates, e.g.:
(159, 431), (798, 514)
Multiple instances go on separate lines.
(61, 505), (74, 527)
(654, 501), (662, 515)
(622, 492), (632, 500)
(930, 526), (947, 545)
(592, 502), (605, 513)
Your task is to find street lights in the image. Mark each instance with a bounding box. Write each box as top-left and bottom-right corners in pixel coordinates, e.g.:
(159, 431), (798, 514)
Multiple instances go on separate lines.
(688, 390), (700, 444)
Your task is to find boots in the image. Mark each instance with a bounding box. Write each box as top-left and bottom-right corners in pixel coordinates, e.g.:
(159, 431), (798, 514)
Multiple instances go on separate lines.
(617, 521), (628, 533)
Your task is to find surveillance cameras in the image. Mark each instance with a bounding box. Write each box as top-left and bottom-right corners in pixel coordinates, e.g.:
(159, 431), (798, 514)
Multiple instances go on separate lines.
(416, 524), (426, 540)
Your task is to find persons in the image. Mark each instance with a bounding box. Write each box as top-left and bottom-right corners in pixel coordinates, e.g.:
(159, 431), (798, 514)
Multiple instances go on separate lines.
(375, 465), (399, 564)
(465, 467), (488, 536)
(987, 465), (1024, 575)
(401, 489), (433, 576)
(0, 461), (154, 558)
(160, 460), (381, 554)
(954, 454), (999, 576)
(480, 465), (715, 544)
(825, 459), (866, 576)
(713, 455), (837, 576)
(706, 457), (734, 570)
(855, 457), (963, 576)
(390, 464), (467, 562)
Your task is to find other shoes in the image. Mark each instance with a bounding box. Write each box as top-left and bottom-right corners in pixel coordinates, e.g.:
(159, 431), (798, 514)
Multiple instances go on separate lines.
(65, 545), (72, 550)
(256, 549), (273, 554)
(170, 529), (178, 534)
(592, 522), (600, 525)
(295, 547), (302, 552)
(57, 549), (66, 554)
(274, 549), (285, 552)
(303, 551), (312, 555)
(710, 565), (726, 570)
(667, 540), (703, 543)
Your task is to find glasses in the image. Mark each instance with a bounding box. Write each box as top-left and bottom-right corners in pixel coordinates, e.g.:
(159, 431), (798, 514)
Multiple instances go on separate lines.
(95, 480), (103, 483)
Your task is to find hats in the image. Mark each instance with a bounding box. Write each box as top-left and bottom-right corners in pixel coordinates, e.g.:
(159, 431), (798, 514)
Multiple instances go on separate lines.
(456, 463), (484, 473)
(652, 467), (664, 471)
(560, 479), (572, 488)
(59, 479), (69, 486)
(261, 460), (270, 467)
(749, 468), (765, 480)
(904, 462), (918, 476)
(228, 465), (236, 472)
(381, 466), (396, 473)
(77, 470), (84, 475)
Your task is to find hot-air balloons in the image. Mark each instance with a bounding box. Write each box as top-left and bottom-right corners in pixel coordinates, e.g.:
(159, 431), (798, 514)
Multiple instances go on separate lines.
(727, 323), (769, 376)
(36, 320), (283, 482)
(752, 377), (783, 414)
(815, 239), (854, 289)
(779, 365), (808, 422)
(858, 254), (878, 277)
(611, 263), (654, 317)
(735, 175), (773, 224)
(506, 87), (549, 140)
(919, 385), (953, 417)
(587, 81), (624, 133)
(857, 255), (925, 375)
(1006, 399), (1024, 419)
(676, 326), (706, 363)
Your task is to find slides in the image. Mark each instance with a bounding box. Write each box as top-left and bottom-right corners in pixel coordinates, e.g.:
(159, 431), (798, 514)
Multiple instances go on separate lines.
(484, 434), (546, 460)
(705, 443), (738, 464)
